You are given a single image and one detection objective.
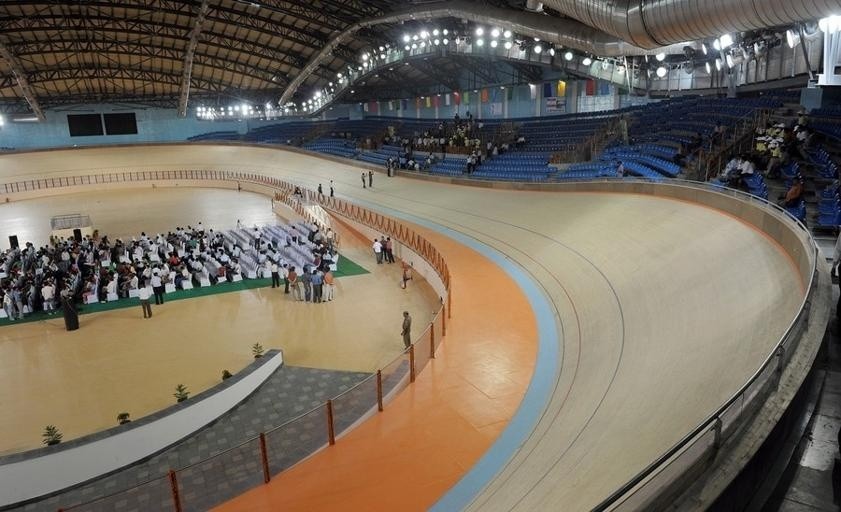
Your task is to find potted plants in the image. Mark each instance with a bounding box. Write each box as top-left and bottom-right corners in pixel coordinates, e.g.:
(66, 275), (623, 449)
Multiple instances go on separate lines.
(116, 412), (131, 425)
(250, 341), (265, 359)
(41, 424), (65, 446)
(171, 384), (191, 402)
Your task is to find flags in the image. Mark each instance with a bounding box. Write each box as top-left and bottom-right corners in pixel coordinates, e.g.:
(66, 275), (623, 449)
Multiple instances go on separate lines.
(357, 79), (610, 116)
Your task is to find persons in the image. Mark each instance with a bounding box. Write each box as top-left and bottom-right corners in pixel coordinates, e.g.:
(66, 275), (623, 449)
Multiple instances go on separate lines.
(617, 160), (624, 178)
(401, 311), (412, 354)
(671, 104), (818, 209)
(329, 179), (336, 197)
(318, 183), (324, 198)
(830, 232), (841, 282)
(361, 172), (367, 188)
(368, 169), (375, 187)
(373, 238), (384, 264)
(380, 236), (389, 262)
(364, 107), (526, 179)
(1, 222), (241, 329)
(386, 236), (396, 264)
(252, 221), (336, 304)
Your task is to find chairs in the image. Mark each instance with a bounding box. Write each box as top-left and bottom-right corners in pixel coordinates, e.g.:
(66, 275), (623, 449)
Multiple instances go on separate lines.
(85, 221), (333, 304)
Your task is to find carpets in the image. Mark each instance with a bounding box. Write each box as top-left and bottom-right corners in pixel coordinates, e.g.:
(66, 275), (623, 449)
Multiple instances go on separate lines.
(0, 246), (372, 325)
